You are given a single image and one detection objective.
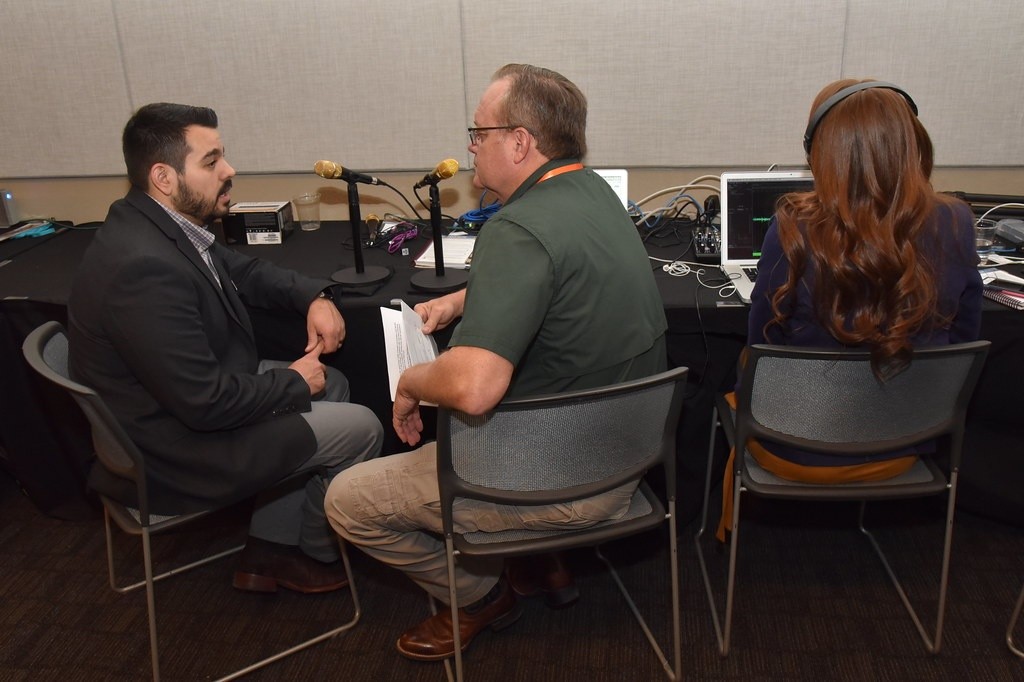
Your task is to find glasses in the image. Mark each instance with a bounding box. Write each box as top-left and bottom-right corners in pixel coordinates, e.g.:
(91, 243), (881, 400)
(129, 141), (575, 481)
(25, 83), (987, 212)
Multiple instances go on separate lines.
(469, 126), (514, 145)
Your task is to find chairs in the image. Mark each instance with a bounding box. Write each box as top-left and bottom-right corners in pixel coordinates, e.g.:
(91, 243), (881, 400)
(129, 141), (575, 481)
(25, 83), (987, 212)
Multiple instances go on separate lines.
(23, 320), (367, 682)
(698, 340), (992, 659)
(426, 366), (691, 682)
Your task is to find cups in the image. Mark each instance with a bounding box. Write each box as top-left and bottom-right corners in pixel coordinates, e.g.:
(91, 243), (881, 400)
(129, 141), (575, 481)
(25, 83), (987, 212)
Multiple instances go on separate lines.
(293, 192), (322, 231)
(973, 218), (998, 266)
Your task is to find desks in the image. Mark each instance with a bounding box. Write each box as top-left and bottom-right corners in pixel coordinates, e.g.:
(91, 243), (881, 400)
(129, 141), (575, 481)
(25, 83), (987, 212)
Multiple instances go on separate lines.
(0, 220), (1024, 530)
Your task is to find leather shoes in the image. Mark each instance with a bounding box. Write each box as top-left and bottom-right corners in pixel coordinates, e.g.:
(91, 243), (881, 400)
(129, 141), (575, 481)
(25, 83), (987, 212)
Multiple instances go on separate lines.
(397, 581), (528, 661)
(508, 554), (577, 603)
(232, 538), (353, 592)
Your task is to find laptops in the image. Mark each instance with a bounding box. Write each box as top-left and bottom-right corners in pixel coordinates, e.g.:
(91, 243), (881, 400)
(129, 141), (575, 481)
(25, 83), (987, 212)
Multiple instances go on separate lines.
(590, 169), (629, 212)
(721, 169), (815, 303)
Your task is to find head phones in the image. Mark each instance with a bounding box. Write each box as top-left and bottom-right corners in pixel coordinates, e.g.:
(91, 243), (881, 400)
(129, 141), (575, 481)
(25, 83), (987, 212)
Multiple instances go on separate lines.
(803, 81), (918, 169)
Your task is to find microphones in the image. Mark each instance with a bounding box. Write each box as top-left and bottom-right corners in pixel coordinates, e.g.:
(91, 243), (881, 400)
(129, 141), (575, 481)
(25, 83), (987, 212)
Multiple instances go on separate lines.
(314, 159), (386, 186)
(413, 159), (459, 189)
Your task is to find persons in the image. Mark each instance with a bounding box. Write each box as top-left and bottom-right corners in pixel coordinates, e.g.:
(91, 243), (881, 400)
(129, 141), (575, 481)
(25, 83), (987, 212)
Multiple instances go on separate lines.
(68, 103), (385, 594)
(715, 80), (984, 543)
(323, 64), (668, 659)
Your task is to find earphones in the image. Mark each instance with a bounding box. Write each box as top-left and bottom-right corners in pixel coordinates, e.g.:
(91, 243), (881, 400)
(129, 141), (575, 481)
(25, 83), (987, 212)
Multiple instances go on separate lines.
(663, 264), (669, 272)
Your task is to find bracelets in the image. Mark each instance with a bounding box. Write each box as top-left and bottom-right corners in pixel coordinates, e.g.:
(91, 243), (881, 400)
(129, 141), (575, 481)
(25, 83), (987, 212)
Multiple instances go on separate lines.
(308, 292), (336, 307)
